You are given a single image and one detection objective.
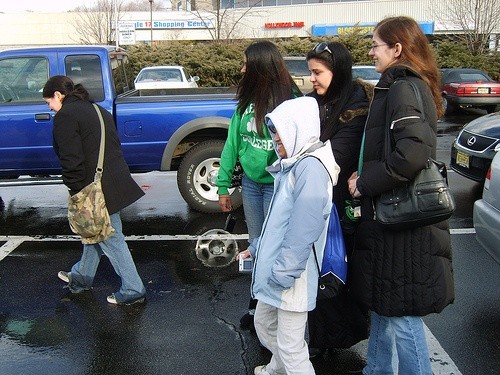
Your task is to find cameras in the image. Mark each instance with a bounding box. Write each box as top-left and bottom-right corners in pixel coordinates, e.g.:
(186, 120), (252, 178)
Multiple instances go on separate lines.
(223, 214), (236, 232)
(238, 254), (254, 273)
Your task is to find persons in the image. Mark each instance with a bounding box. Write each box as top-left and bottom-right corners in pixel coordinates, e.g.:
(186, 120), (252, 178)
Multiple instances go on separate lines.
(236, 96), (340, 375)
(348, 17), (456, 375)
(217, 40), (304, 336)
(304, 41), (374, 360)
(43, 76), (147, 306)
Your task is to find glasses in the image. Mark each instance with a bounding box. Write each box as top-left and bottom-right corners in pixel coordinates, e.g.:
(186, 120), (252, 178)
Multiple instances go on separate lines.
(368, 42), (397, 49)
(312, 42), (333, 55)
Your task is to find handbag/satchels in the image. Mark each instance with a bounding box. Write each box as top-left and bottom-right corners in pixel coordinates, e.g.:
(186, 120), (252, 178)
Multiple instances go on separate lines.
(67, 184), (115, 244)
(375, 159), (457, 228)
(229, 162), (242, 188)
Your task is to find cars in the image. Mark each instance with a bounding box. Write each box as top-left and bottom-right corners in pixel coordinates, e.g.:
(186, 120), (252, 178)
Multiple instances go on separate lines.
(439, 69), (500, 120)
(472, 152), (500, 267)
(449, 111), (500, 183)
(351, 65), (382, 86)
(132, 66), (199, 89)
(282, 55), (315, 96)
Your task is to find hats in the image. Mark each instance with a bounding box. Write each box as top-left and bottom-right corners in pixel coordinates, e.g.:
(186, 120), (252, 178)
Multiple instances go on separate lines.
(267, 120), (276, 134)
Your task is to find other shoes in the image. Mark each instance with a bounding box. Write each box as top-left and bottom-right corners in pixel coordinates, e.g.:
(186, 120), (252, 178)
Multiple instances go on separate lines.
(254, 365), (271, 375)
(240, 309), (253, 326)
(107, 294), (145, 305)
(348, 370), (363, 375)
(309, 346), (323, 358)
(58, 271), (69, 283)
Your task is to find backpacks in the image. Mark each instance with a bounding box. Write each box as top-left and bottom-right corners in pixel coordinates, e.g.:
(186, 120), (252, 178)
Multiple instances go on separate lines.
(294, 156), (348, 298)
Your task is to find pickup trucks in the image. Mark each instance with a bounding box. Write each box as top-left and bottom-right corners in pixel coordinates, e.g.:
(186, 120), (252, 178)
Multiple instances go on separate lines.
(0, 47), (243, 214)
